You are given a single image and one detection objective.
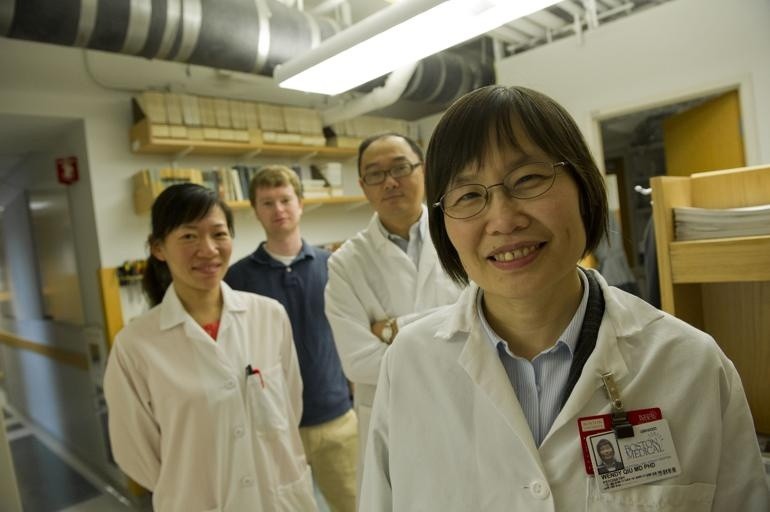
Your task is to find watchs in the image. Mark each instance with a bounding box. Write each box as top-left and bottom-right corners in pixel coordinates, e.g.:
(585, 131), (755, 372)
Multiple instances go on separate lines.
(381, 316), (397, 346)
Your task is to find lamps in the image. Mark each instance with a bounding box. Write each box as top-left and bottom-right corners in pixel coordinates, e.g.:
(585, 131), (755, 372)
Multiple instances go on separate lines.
(270, 0), (565, 96)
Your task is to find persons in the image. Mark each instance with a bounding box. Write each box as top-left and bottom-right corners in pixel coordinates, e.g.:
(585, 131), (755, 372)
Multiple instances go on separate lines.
(322, 129), (471, 453)
(356, 83), (769, 512)
(101, 182), (323, 512)
(594, 436), (625, 477)
(221, 161), (361, 512)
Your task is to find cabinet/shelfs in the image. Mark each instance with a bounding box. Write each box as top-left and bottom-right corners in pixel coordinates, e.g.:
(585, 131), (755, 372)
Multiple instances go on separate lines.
(648, 164), (770, 458)
(131, 96), (372, 216)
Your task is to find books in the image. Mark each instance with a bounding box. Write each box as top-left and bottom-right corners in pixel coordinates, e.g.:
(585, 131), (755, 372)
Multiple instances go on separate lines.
(670, 203), (770, 244)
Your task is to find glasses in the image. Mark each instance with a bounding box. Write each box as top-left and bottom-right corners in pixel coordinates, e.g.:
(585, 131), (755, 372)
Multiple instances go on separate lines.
(432, 161), (568, 221)
(359, 161), (421, 185)
